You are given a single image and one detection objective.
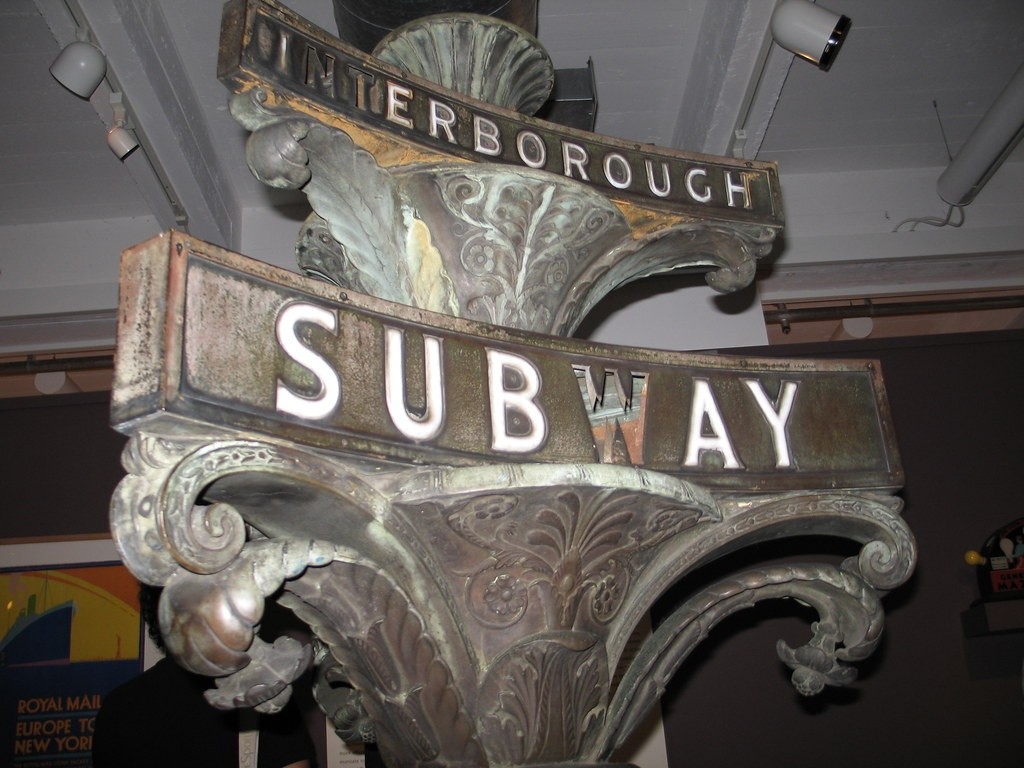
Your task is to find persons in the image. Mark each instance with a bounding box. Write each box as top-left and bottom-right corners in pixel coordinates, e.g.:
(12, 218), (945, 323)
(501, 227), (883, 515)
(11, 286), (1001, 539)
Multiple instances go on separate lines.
(89, 582), (319, 768)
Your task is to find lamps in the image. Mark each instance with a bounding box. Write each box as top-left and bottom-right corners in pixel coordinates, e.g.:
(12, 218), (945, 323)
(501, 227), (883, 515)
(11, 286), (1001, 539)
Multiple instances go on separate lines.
(50, 28), (105, 99)
(771, 1), (852, 73)
(105, 90), (137, 162)
(933, 63), (1024, 205)
